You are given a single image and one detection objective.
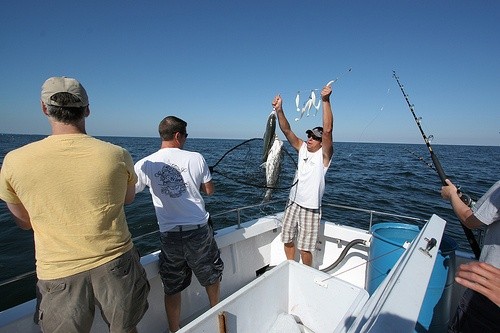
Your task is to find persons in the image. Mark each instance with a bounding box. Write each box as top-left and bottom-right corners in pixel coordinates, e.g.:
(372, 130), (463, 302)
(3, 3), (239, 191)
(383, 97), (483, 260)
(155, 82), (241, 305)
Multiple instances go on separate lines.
(272, 85), (333, 267)
(0, 77), (150, 333)
(133, 116), (224, 333)
(441, 178), (500, 333)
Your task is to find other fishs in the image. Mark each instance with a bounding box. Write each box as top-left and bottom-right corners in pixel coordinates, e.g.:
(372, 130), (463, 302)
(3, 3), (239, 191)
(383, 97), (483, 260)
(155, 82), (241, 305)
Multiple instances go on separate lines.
(260, 111), (286, 204)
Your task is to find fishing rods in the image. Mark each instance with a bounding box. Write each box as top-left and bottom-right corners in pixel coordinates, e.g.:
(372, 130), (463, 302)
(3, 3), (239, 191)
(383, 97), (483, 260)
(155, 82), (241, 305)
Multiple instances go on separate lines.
(393, 70), (481, 261)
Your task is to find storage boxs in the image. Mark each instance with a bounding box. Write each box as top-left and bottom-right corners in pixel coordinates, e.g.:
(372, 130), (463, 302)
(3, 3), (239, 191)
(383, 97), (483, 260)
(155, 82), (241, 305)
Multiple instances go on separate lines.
(174, 214), (447, 333)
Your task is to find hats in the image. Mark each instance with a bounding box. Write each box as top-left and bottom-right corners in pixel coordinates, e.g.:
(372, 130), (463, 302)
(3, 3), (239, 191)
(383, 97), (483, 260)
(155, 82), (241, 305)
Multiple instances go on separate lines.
(40, 76), (91, 109)
(305, 126), (323, 138)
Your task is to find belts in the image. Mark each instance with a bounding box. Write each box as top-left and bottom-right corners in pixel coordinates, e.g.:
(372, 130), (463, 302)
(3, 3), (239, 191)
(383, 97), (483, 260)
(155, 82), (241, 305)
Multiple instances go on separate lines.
(165, 222), (208, 232)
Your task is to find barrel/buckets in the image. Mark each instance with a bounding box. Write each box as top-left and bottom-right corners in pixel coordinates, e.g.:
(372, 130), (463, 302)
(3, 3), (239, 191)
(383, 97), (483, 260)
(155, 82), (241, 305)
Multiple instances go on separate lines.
(366, 223), (458, 333)
